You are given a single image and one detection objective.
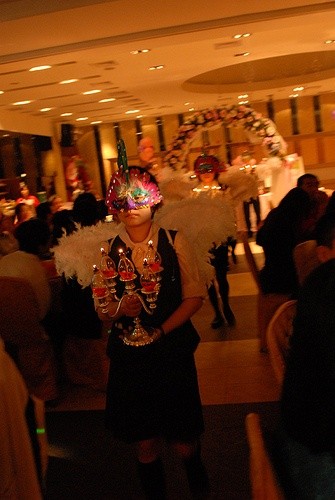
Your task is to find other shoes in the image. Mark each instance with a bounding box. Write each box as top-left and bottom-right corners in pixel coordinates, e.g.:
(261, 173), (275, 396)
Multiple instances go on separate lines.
(212, 313), (223, 328)
(223, 308), (235, 326)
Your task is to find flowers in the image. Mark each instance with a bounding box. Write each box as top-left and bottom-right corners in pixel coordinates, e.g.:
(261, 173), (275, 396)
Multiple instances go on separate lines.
(160, 103), (288, 168)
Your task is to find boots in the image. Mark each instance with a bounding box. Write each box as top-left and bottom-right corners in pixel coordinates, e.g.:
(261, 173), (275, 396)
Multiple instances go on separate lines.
(137, 458), (165, 500)
(184, 441), (209, 492)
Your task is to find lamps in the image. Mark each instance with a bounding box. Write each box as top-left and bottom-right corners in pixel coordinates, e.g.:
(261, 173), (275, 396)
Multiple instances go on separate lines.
(91, 239), (164, 347)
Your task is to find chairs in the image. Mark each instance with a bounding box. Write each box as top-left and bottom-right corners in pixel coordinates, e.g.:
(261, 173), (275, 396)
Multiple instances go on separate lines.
(63, 315), (113, 391)
(266, 300), (296, 382)
(245, 412), (288, 500)
(241, 233), (288, 351)
(0, 274), (61, 402)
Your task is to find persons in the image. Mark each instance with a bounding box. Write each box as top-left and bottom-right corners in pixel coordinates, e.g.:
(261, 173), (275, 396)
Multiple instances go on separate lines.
(0, 159), (107, 260)
(53, 139), (237, 500)
(160, 154), (264, 328)
(244, 173), (335, 500)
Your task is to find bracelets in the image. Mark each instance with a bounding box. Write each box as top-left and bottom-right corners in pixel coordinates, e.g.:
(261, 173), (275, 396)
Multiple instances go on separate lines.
(155, 326), (164, 343)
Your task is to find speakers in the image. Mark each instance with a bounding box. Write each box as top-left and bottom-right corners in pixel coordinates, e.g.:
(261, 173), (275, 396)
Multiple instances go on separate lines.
(61, 124), (73, 147)
